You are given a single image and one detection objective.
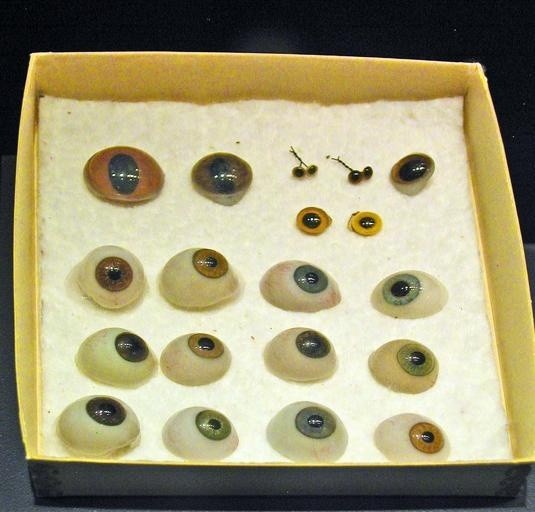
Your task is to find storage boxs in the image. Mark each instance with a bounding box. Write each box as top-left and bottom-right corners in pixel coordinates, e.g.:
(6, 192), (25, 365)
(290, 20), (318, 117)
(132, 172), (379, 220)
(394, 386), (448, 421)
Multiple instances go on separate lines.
(11, 52), (535, 504)
